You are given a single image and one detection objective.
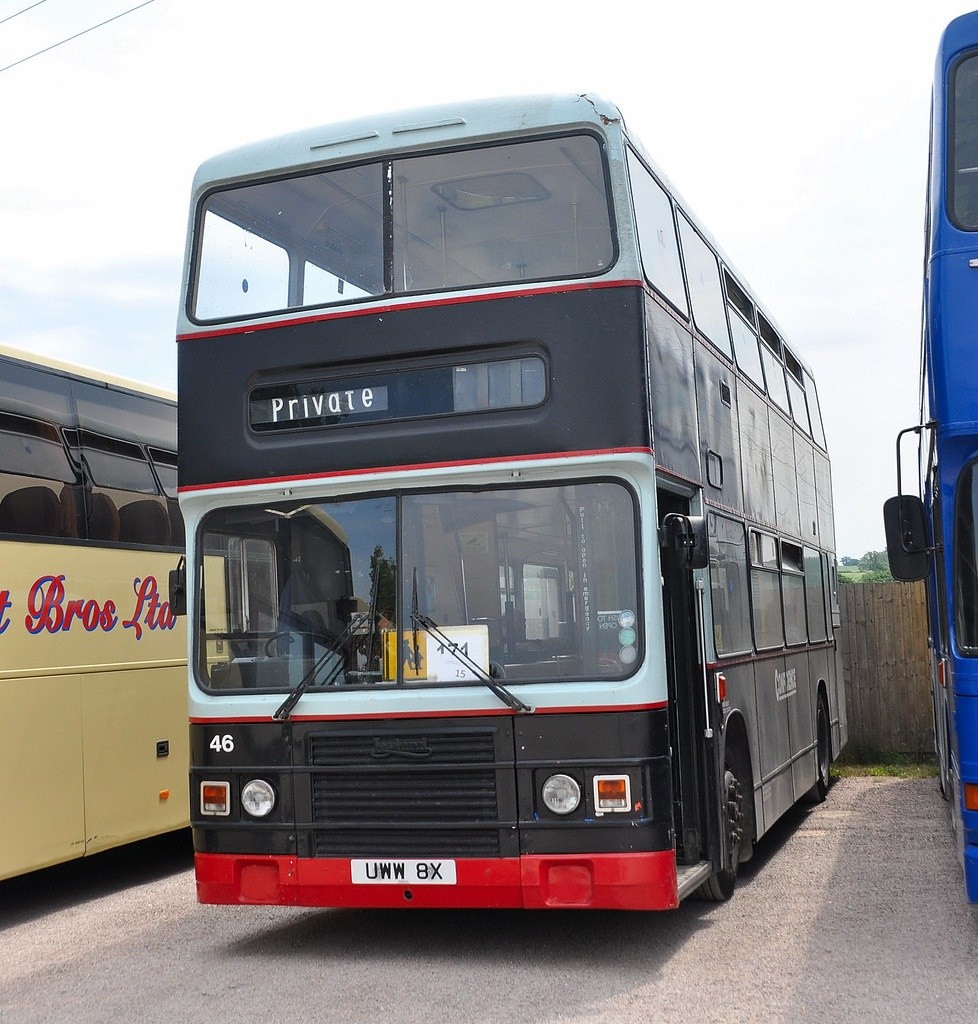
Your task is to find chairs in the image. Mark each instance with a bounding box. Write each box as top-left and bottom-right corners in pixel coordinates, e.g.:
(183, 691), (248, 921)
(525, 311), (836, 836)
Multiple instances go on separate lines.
(0, 487), (173, 546)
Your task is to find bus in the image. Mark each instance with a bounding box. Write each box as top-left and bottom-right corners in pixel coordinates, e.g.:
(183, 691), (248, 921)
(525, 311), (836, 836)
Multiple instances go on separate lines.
(0, 345), (276, 895)
(168, 89), (850, 925)
(880, 10), (978, 906)
